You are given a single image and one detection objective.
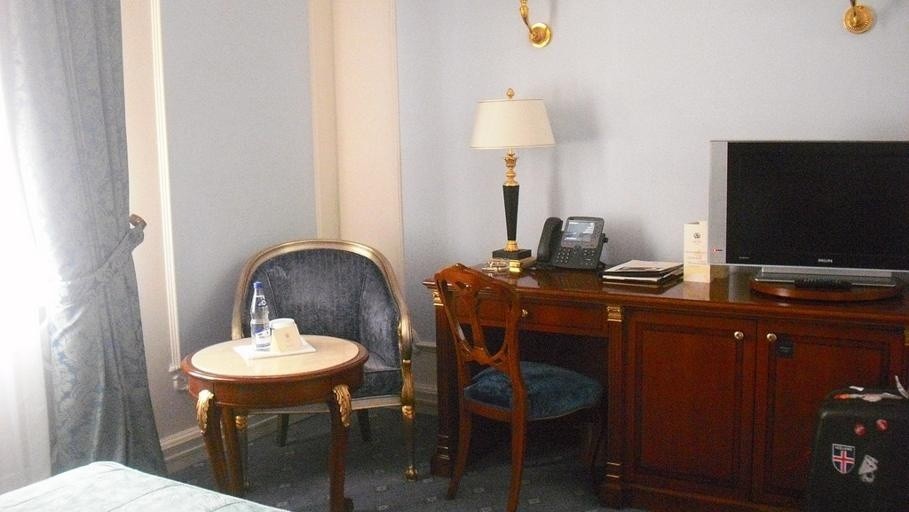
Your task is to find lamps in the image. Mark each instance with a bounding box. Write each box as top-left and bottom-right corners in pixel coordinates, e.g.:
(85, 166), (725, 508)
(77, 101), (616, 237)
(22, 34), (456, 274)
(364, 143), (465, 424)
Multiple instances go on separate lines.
(467, 86), (556, 272)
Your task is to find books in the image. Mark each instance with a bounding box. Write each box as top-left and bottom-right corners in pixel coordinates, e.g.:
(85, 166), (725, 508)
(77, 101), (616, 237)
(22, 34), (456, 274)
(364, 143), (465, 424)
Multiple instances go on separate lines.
(600, 259), (683, 282)
(600, 273), (682, 290)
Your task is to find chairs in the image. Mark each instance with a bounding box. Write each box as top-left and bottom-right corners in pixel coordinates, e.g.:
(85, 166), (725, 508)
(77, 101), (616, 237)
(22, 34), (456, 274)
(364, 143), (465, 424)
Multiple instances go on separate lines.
(433, 262), (607, 511)
(228, 238), (417, 490)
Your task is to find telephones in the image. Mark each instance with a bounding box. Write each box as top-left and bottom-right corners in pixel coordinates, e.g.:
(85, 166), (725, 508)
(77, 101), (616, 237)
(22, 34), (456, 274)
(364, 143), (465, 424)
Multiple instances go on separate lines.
(536, 217), (608, 271)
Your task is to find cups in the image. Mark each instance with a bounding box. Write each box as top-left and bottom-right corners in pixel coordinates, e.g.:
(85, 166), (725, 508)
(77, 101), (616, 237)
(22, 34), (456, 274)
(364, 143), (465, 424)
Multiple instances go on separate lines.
(267, 317), (301, 352)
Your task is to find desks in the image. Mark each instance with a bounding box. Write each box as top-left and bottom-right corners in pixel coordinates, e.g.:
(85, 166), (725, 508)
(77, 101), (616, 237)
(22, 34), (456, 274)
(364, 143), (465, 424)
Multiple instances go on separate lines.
(181, 335), (369, 512)
(421, 257), (909, 512)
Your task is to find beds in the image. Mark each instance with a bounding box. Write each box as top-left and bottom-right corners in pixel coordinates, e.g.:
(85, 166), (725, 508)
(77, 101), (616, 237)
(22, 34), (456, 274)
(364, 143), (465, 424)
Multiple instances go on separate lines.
(0, 460), (294, 512)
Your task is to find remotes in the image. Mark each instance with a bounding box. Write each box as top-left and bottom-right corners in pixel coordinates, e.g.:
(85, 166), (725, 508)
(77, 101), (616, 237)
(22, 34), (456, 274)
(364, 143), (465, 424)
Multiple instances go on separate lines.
(793, 277), (852, 289)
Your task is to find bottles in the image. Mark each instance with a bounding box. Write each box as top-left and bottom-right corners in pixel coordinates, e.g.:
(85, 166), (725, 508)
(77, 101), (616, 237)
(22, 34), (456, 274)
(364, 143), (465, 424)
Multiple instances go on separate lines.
(249, 281), (271, 352)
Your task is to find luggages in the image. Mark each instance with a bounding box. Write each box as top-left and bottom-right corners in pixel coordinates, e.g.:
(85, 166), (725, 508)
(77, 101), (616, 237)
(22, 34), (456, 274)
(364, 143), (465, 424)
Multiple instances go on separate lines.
(807, 386), (909, 512)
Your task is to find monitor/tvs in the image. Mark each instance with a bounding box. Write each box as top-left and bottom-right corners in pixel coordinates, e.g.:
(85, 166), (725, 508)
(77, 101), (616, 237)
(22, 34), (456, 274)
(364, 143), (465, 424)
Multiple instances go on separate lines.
(706, 139), (909, 287)
